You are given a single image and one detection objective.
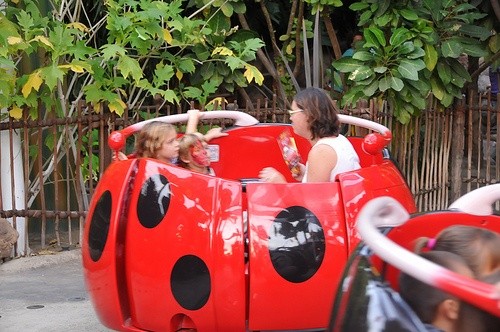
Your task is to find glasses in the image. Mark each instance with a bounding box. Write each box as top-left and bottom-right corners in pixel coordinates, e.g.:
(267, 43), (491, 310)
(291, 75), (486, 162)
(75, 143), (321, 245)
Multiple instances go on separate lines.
(287, 109), (305, 114)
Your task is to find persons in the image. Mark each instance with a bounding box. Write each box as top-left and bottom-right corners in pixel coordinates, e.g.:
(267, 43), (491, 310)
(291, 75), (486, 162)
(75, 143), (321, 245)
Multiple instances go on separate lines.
(258, 88), (361, 183)
(399, 250), (475, 331)
(415, 225), (500, 281)
(112, 109), (229, 176)
